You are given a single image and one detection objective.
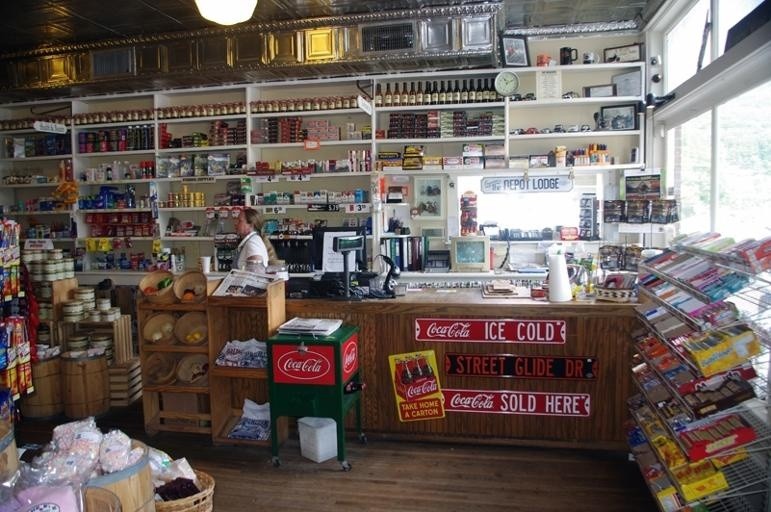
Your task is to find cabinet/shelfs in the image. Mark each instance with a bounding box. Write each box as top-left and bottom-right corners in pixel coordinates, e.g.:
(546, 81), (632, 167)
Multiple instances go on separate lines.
(0, 62), (650, 286)
(631, 244), (769, 511)
(29, 277), (288, 450)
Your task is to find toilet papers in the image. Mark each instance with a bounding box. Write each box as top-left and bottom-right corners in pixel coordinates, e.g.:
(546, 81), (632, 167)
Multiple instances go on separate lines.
(547, 254), (573, 302)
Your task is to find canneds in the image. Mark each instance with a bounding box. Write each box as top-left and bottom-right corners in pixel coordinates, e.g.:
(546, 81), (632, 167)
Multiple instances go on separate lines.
(158, 184), (205, 207)
(24, 249), (121, 360)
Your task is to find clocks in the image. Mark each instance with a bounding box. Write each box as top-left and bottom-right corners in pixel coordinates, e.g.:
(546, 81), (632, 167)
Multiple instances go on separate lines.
(493, 70), (520, 97)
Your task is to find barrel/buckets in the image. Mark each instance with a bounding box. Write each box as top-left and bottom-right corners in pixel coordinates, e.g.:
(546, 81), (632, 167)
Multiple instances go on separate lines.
(0, 270), (217, 512)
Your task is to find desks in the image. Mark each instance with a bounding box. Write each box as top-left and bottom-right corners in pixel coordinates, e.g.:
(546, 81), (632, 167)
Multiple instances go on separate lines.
(264, 323), (362, 460)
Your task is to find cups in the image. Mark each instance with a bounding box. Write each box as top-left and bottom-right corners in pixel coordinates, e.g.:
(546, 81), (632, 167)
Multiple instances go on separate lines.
(560, 47), (578, 65)
(537, 55), (552, 68)
(198, 257), (211, 276)
(583, 51), (600, 64)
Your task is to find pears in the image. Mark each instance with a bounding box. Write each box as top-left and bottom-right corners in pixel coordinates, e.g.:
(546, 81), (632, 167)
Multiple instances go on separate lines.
(186, 332), (200, 343)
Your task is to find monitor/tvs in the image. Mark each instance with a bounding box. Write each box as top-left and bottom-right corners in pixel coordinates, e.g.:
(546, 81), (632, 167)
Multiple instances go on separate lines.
(450, 236), (490, 272)
(314, 226), (369, 271)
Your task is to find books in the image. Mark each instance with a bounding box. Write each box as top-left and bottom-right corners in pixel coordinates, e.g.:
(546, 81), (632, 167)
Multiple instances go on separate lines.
(383, 238), (423, 271)
(483, 284), (519, 296)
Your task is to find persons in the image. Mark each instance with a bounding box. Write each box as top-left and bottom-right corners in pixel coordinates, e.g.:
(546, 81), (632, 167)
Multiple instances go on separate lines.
(234, 208), (276, 270)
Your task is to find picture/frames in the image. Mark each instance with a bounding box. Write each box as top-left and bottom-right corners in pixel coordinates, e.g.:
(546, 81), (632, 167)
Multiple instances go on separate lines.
(603, 43), (641, 62)
(498, 33), (531, 67)
(601, 104), (638, 130)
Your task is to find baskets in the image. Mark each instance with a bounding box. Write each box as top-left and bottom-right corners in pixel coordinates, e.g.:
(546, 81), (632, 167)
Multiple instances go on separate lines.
(154, 467), (216, 512)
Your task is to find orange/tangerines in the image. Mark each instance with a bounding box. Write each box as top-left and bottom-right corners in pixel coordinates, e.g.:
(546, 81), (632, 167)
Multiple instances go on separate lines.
(183, 293), (193, 299)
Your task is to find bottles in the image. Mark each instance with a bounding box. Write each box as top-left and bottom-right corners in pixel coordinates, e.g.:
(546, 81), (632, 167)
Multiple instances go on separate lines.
(106, 164), (113, 181)
(59, 159), (71, 183)
(0, 94), (369, 130)
(375, 79), (503, 106)
(126, 125), (155, 150)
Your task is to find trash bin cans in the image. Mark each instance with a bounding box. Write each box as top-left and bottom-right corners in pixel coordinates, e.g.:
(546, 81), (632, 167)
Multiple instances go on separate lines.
(297, 417), (338, 464)
